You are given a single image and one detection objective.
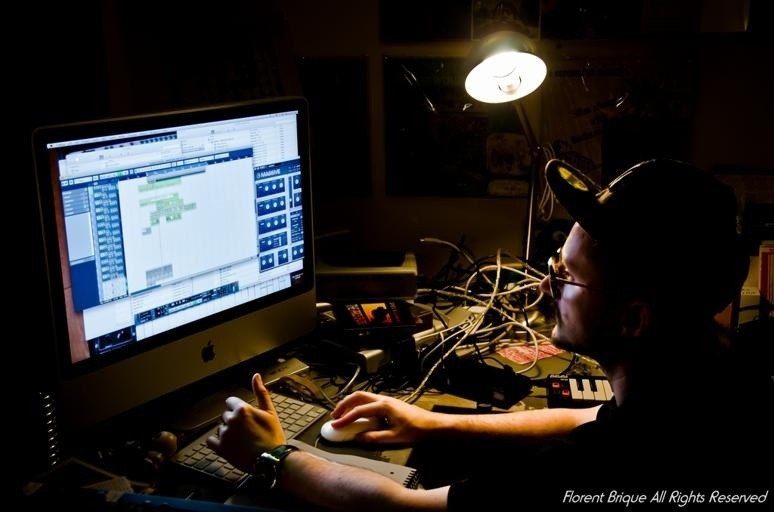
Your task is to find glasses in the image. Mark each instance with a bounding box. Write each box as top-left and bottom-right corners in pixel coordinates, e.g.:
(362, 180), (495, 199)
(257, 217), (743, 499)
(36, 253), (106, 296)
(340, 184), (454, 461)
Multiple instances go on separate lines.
(547, 246), (591, 299)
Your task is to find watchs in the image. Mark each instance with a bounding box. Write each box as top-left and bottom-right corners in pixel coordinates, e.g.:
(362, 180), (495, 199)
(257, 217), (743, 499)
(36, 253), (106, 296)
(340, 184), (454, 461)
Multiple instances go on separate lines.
(246, 444), (306, 484)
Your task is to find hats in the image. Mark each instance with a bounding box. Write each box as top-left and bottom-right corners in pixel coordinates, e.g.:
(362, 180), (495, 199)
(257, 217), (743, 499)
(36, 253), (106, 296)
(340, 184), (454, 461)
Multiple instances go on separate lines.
(543, 155), (751, 316)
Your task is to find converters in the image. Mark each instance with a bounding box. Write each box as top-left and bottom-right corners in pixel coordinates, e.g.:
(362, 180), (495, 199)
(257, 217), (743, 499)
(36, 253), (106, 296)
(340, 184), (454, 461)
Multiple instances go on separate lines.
(355, 347), (389, 376)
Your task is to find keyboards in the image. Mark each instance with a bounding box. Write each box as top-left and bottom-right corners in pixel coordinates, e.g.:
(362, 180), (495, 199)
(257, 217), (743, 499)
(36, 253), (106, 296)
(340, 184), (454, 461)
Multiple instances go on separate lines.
(169, 390), (328, 487)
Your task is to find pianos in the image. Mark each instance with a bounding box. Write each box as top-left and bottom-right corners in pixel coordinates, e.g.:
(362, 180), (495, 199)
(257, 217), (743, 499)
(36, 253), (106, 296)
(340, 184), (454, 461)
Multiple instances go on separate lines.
(547, 373), (614, 408)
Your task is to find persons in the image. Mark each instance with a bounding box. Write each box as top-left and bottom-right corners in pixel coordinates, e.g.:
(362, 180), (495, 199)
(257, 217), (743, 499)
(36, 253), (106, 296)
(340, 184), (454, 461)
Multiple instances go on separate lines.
(201, 154), (773, 511)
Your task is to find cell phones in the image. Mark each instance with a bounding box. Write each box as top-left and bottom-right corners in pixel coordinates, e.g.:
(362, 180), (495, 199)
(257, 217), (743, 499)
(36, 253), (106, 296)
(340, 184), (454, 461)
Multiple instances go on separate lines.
(332, 298), (413, 330)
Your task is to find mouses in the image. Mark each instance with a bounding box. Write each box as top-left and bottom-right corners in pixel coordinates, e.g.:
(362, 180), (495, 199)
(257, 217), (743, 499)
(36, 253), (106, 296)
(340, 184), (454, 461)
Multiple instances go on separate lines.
(320, 414), (386, 445)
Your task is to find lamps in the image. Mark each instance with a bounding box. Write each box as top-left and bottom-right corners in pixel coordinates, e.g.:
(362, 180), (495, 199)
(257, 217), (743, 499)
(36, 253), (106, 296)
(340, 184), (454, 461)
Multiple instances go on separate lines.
(463, 30), (548, 329)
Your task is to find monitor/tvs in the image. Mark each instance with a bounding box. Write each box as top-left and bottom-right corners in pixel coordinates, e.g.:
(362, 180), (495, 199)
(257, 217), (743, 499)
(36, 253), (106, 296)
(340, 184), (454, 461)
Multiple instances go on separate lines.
(31, 95), (319, 434)
